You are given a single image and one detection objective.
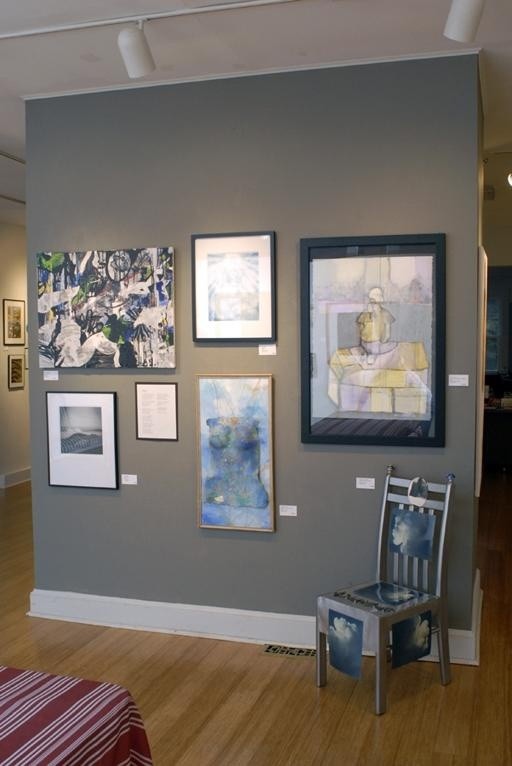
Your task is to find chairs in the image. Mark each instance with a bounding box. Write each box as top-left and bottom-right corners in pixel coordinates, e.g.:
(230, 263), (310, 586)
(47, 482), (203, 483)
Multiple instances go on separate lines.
(315, 465), (456, 717)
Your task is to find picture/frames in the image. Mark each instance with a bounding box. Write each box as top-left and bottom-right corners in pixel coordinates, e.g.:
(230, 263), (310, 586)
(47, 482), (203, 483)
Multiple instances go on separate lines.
(8, 356), (25, 390)
(4, 299), (26, 346)
(299, 234), (446, 445)
(194, 372), (277, 534)
(47, 392), (121, 491)
(193, 233), (276, 342)
(35, 247), (179, 370)
